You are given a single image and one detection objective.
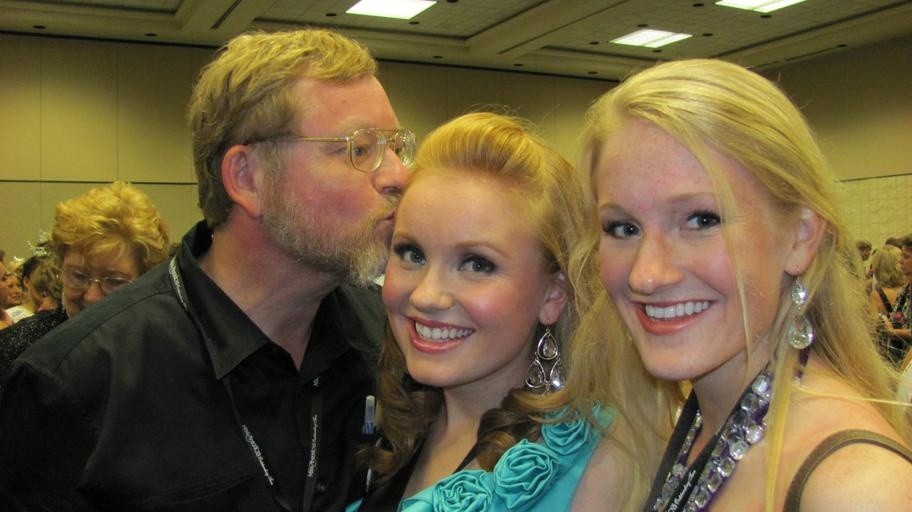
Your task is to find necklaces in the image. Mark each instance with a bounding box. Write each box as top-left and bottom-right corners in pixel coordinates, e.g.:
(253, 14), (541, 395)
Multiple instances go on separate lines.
(653, 354), (805, 511)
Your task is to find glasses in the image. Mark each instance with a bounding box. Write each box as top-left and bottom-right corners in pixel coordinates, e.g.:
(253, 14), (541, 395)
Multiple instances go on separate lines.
(291, 125), (416, 173)
(56, 267), (132, 298)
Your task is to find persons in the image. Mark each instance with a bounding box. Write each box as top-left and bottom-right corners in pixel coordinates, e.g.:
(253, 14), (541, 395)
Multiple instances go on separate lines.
(383, 105), (637, 512)
(565, 52), (912, 512)
(0, 26), (446, 511)
(853, 231), (912, 366)
(0, 179), (172, 375)
